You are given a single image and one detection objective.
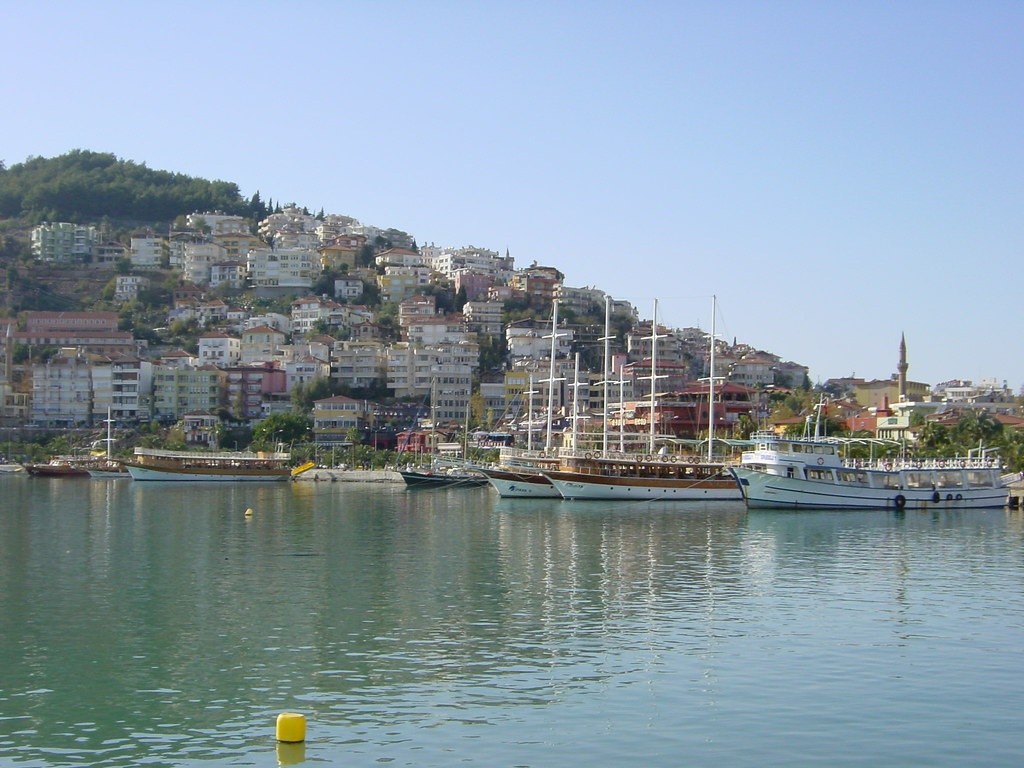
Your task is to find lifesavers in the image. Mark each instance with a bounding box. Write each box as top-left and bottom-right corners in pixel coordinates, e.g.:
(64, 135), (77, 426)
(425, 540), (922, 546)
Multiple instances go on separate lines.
(636, 455), (643, 462)
(938, 459), (945, 468)
(646, 455), (652, 462)
(960, 461), (966, 468)
(956, 494), (962, 500)
(670, 455), (677, 463)
(895, 494), (906, 507)
(916, 461), (922, 468)
(947, 494), (953, 500)
(986, 460), (992, 467)
(694, 456), (700, 464)
(539, 451), (546, 458)
(584, 452), (592, 460)
(662, 455), (668, 462)
(884, 462), (892, 471)
(594, 451), (601, 459)
(688, 456), (694, 464)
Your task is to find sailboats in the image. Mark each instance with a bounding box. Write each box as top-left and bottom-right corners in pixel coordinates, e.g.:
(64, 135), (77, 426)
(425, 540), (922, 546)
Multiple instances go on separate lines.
(383, 295), (1014, 511)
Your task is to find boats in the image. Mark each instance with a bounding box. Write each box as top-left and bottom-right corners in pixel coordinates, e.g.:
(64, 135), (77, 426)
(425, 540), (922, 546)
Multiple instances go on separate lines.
(16, 406), (294, 484)
